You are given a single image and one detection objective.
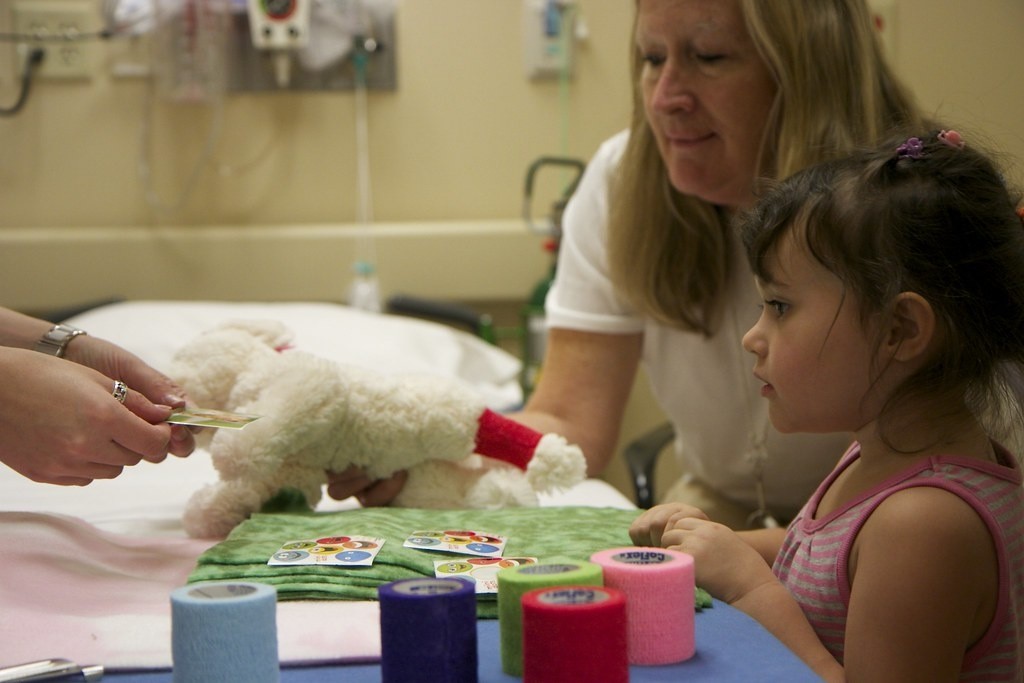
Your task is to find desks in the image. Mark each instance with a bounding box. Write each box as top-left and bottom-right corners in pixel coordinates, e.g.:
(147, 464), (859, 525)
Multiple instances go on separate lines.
(0, 597), (822, 683)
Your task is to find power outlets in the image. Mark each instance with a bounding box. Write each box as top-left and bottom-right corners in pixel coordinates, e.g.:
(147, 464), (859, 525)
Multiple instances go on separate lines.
(16, 2), (98, 78)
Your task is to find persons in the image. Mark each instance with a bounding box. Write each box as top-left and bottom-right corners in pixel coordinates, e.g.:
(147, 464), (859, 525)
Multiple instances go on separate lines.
(631, 130), (1024, 683)
(0, 302), (205, 486)
(328, 0), (1024, 533)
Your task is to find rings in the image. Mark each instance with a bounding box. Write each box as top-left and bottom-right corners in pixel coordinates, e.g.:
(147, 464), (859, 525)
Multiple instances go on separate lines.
(112, 380), (130, 404)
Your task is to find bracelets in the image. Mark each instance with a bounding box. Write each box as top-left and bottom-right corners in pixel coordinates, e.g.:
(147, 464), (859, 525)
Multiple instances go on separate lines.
(33, 323), (87, 358)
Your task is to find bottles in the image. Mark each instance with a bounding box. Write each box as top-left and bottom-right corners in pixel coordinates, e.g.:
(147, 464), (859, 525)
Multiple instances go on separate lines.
(350, 261), (381, 311)
(522, 242), (560, 399)
